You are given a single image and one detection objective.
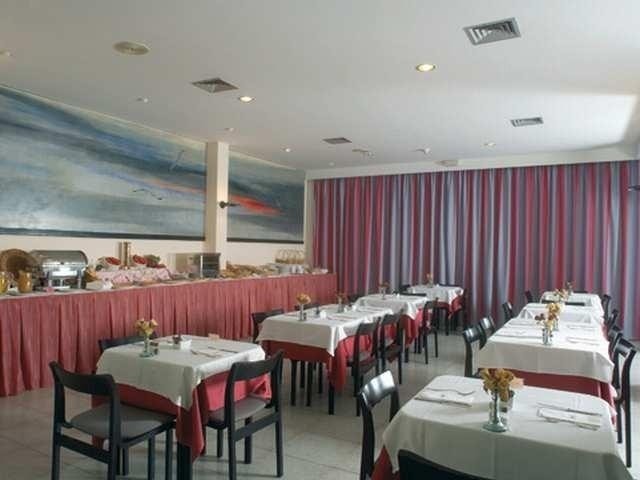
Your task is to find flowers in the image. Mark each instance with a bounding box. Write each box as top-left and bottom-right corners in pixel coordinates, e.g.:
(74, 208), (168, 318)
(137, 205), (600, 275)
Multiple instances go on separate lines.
(133, 318), (158, 337)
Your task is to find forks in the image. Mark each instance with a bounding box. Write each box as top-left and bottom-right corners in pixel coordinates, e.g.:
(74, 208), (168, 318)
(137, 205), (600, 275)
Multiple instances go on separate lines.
(151, 306), (357, 371)
(410, 278), (603, 432)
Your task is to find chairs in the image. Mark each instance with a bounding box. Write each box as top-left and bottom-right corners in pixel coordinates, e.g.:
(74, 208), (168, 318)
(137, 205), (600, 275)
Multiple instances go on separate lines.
(203, 350), (284, 480)
(357, 372), (400, 480)
(251, 283), (466, 414)
(601, 294), (637, 468)
(460, 289), (636, 467)
(50, 361), (174, 480)
(99, 331), (157, 353)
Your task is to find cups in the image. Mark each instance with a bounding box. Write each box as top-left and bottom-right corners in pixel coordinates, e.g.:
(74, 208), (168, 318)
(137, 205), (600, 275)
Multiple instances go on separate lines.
(277, 249), (305, 265)
(0, 268), (34, 295)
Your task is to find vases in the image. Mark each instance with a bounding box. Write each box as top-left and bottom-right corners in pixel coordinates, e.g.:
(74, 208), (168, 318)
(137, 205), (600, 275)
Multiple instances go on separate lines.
(140, 337), (152, 357)
